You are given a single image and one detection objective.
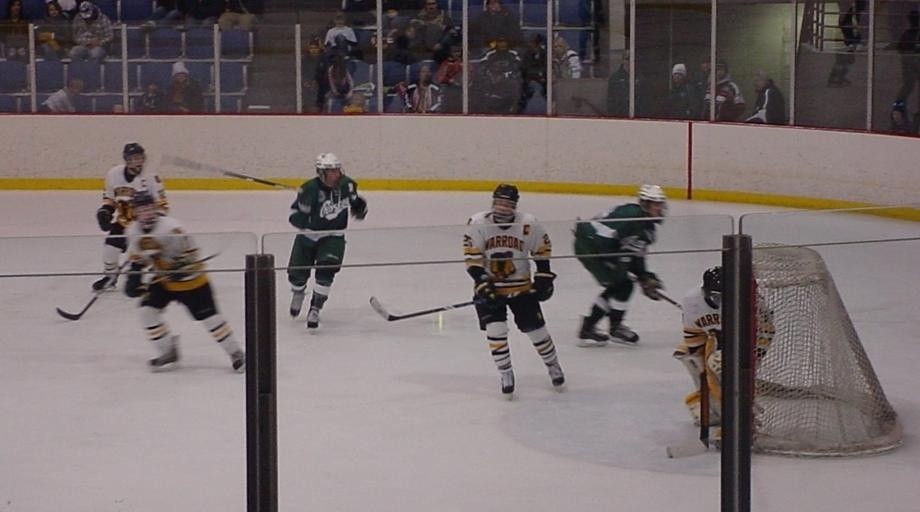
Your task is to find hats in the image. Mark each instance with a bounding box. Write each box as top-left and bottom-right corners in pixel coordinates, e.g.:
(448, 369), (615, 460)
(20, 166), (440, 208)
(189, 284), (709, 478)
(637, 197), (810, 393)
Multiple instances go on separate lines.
(335, 11), (345, 18)
(672, 64), (687, 78)
(77, 0), (93, 13)
(497, 33), (506, 41)
(171, 62), (189, 78)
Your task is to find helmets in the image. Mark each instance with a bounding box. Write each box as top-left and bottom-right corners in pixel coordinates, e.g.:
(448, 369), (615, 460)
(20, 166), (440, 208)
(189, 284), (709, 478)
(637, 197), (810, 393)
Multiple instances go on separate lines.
(123, 143), (146, 161)
(890, 100), (907, 120)
(129, 194), (154, 208)
(639, 184), (667, 203)
(315, 152), (342, 171)
(701, 265), (721, 310)
(492, 183), (520, 205)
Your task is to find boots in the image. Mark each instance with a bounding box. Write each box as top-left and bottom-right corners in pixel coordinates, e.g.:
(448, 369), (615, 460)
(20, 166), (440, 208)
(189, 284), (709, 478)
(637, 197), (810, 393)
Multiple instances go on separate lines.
(289, 285), (308, 318)
(578, 303), (609, 342)
(609, 308), (639, 343)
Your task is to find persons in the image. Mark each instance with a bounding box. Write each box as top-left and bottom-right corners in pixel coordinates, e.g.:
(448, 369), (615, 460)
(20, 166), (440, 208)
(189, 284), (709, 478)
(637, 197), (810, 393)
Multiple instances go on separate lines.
(92, 142), (170, 293)
(122, 195), (247, 375)
(825, 0), (868, 89)
(464, 182), (565, 399)
(886, 11), (920, 99)
(674, 266), (775, 427)
(888, 101), (914, 136)
(574, 182), (667, 346)
(283, 152), (368, 334)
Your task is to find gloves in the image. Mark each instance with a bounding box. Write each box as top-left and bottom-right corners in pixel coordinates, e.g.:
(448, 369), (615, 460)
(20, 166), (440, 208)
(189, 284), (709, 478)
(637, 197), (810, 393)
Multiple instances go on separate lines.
(95, 207), (114, 232)
(349, 197), (368, 219)
(530, 270), (557, 302)
(473, 277), (496, 304)
(636, 272), (664, 301)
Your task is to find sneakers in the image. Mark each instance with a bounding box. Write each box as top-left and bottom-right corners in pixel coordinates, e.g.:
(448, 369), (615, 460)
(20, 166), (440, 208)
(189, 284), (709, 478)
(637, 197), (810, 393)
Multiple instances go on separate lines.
(827, 77), (852, 88)
(306, 291), (328, 328)
(229, 348), (245, 372)
(544, 360), (565, 387)
(146, 347), (179, 367)
(497, 366), (515, 394)
(92, 274), (118, 290)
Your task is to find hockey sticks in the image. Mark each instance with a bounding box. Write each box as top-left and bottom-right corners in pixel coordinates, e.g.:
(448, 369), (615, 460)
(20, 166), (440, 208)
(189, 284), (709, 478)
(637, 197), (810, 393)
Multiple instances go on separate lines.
(56, 261), (126, 321)
(370, 287), (536, 322)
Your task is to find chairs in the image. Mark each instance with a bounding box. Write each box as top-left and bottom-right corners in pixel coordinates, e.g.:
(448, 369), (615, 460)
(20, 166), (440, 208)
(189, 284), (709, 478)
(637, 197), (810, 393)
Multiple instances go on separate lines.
(320, 1), (600, 114)
(1, 0), (262, 116)
